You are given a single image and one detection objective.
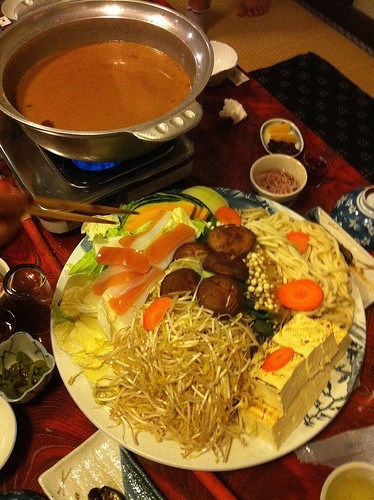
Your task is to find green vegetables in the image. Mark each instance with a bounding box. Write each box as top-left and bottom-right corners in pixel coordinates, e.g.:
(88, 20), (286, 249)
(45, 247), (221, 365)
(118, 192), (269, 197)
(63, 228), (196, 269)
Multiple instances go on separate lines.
(0, 351), (50, 399)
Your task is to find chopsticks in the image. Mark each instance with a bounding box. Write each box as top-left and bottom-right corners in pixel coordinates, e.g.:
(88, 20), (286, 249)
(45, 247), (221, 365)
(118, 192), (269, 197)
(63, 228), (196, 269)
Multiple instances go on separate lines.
(25, 195), (140, 225)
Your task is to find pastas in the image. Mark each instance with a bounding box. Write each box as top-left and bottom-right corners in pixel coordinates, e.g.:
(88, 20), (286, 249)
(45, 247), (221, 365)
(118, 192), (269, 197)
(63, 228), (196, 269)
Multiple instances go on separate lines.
(236, 209), (355, 333)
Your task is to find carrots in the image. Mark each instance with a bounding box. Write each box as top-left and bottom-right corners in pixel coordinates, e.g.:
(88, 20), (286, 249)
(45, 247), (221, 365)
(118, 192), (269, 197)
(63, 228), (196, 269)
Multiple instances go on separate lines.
(261, 346), (294, 370)
(143, 296), (174, 331)
(277, 280), (325, 311)
(286, 230), (309, 253)
(215, 207), (242, 225)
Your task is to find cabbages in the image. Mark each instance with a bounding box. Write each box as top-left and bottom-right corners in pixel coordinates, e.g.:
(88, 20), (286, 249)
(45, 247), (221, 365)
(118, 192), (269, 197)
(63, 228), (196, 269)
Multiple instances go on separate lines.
(52, 193), (215, 388)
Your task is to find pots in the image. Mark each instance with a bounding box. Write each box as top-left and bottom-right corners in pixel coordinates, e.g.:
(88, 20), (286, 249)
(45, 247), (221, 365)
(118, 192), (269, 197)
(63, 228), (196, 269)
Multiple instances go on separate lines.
(0, 0), (215, 160)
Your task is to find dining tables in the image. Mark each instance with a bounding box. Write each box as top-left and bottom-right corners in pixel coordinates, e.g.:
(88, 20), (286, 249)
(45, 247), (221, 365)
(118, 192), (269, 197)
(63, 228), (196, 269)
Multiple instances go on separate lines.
(1, 0), (373, 500)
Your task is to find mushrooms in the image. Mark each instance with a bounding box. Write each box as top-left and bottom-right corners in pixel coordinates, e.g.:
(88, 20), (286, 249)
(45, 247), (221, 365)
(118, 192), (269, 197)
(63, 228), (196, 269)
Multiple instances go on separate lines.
(160, 225), (257, 320)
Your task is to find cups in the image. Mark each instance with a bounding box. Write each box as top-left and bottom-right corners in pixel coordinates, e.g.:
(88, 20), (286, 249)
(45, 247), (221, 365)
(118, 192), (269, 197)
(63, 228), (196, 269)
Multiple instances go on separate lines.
(0, 331), (55, 403)
(0, 309), (16, 343)
(3, 264), (54, 336)
(320, 461), (374, 500)
(0, 258), (12, 309)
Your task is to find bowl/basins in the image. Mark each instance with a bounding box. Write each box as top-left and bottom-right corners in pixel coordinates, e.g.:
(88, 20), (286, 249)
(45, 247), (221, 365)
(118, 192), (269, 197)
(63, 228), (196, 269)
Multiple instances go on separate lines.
(0, 396), (17, 469)
(207, 41), (239, 86)
(249, 154), (308, 202)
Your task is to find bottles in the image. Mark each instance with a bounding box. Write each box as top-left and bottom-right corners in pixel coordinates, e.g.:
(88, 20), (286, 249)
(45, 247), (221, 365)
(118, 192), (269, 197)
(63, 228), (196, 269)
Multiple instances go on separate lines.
(330, 185), (374, 251)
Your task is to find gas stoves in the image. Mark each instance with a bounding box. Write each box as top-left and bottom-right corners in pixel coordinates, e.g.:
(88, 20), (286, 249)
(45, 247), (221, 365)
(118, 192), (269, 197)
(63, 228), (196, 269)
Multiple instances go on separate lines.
(0, 112), (215, 234)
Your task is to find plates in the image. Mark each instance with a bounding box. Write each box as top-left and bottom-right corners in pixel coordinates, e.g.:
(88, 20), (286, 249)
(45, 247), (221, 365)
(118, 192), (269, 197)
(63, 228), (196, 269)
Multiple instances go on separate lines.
(1, 1), (33, 21)
(37, 429), (165, 500)
(51, 187), (367, 471)
(0, 490), (49, 500)
(302, 205), (374, 310)
(260, 118), (304, 157)
